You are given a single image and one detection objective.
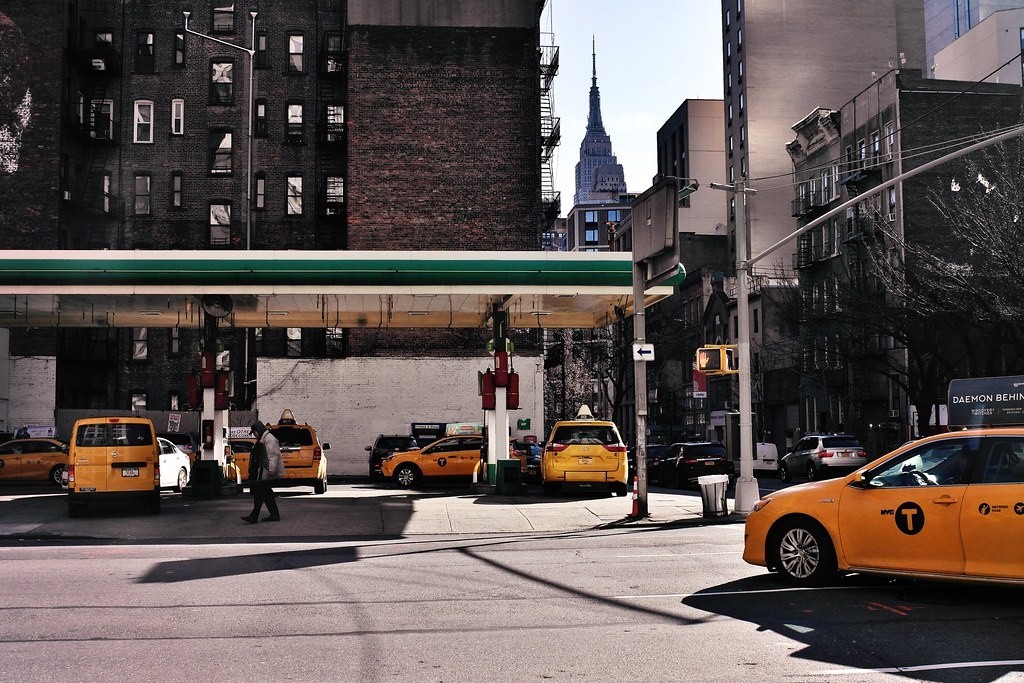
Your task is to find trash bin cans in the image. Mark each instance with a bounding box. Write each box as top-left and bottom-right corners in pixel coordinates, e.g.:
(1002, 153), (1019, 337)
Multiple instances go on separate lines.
(698, 474), (730, 519)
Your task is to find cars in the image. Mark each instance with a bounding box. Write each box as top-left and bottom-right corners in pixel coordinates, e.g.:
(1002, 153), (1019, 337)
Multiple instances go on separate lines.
(538, 405), (629, 496)
(261, 408), (332, 495)
(628, 444), (667, 472)
(381, 423), (529, 489)
(62, 436), (191, 493)
(186, 438), (262, 479)
(741, 426), (1024, 594)
(0, 438), (71, 486)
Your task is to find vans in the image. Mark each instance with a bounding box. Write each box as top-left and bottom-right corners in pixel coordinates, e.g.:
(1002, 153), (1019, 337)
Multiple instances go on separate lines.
(60, 417), (161, 518)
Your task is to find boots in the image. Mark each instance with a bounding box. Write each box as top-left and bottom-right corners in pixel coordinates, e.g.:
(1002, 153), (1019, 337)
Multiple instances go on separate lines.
(241, 510), (258, 523)
(261, 511), (280, 521)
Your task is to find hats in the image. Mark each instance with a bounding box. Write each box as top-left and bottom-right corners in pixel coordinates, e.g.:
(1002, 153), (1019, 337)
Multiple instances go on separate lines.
(248, 420), (265, 436)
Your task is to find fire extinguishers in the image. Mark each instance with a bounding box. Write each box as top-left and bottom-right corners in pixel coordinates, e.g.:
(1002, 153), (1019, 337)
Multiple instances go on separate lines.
(506, 368), (520, 409)
(494, 346), (508, 387)
(481, 369), (496, 410)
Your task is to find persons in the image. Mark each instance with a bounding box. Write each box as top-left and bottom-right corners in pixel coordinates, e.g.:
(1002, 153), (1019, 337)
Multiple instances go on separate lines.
(240, 420), (287, 524)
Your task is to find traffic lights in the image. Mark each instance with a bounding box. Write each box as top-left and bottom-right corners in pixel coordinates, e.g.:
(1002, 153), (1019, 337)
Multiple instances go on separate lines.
(695, 348), (724, 372)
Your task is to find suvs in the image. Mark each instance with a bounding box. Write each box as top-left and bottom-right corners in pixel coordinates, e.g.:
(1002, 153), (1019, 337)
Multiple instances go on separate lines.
(777, 434), (868, 481)
(646, 440), (735, 488)
(364, 433), (419, 483)
